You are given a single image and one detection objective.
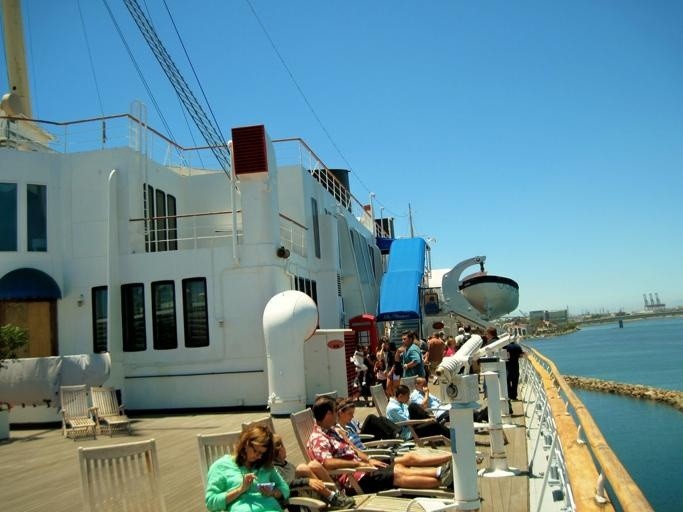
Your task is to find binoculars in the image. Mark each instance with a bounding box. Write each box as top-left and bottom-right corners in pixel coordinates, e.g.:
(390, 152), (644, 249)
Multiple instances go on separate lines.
(406, 331), (519, 511)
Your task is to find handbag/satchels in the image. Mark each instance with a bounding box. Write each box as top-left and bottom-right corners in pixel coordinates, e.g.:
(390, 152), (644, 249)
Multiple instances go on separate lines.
(376, 359), (385, 371)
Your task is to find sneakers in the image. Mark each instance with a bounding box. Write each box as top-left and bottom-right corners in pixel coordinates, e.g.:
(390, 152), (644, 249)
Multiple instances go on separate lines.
(438, 456), (453, 486)
(329, 491), (355, 509)
(366, 401), (375, 407)
(432, 375), (439, 385)
(355, 376), (367, 387)
(508, 397), (518, 402)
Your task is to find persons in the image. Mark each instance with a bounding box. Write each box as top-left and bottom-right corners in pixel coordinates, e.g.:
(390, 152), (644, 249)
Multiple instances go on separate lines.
(385, 384), (450, 441)
(305, 395), (455, 496)
(350, 413), (415, 449)
(424, 296), (439, 314)
(202, 423), (291, 512)
(267, 433), (356, 512)
(331, 396), (451, 470)
(351, 323), (527, 423)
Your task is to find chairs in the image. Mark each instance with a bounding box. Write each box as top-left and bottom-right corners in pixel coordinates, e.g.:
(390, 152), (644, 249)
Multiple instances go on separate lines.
(60, 385), (134, 440)
(195, 375), (447, 512)
(77, 438), (167, 512)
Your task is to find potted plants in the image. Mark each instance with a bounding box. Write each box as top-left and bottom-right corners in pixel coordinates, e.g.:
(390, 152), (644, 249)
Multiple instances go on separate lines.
(0, 324), (31, 441)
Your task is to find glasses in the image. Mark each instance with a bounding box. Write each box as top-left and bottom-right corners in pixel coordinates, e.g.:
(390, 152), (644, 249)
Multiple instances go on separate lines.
(251, 445), (266, 455)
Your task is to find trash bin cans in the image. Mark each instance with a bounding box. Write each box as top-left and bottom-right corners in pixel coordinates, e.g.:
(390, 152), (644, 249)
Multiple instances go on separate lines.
(0, 401), (11, 439)
